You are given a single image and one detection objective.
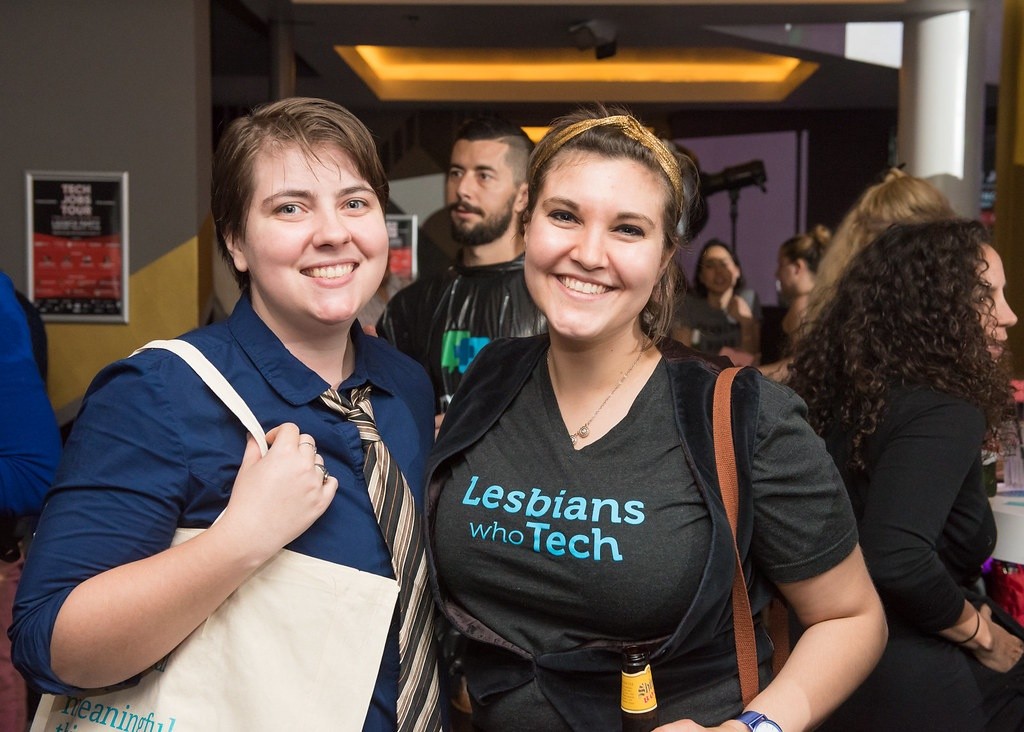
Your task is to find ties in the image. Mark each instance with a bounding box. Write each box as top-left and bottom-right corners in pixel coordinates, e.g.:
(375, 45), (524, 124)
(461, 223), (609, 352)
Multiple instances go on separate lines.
(320, 383), (442, 732)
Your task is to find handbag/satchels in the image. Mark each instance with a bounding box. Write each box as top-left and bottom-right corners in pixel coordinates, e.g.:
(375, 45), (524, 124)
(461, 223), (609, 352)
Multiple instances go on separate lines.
(28, 339), (400, 732)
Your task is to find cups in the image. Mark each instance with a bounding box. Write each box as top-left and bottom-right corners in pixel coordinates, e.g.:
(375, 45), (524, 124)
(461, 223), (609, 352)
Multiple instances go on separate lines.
(982, 451), (998, 497)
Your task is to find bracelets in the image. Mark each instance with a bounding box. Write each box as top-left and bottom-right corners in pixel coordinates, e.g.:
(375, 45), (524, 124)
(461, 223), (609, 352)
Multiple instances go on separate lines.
(957, 612), (979, 645)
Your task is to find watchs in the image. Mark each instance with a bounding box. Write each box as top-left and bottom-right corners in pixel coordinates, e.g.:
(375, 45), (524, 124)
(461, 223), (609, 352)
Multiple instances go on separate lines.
(736, 710), (783, 732)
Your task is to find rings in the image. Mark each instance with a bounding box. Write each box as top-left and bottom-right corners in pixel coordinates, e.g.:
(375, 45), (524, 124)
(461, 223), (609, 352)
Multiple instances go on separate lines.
(314, 464), (329, 486)
(299, 442), (317, 454)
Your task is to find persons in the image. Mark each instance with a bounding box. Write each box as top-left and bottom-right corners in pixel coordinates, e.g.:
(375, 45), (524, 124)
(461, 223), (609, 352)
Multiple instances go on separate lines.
(1, 98), (1023, 732)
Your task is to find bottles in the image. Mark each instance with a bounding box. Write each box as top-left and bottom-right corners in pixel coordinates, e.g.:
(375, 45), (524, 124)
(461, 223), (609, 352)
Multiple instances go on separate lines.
(618, 644), (659, 732)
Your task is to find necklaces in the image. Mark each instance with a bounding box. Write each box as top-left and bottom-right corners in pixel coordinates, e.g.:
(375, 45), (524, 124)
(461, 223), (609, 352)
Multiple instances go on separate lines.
(546, 338), (645, 446)
